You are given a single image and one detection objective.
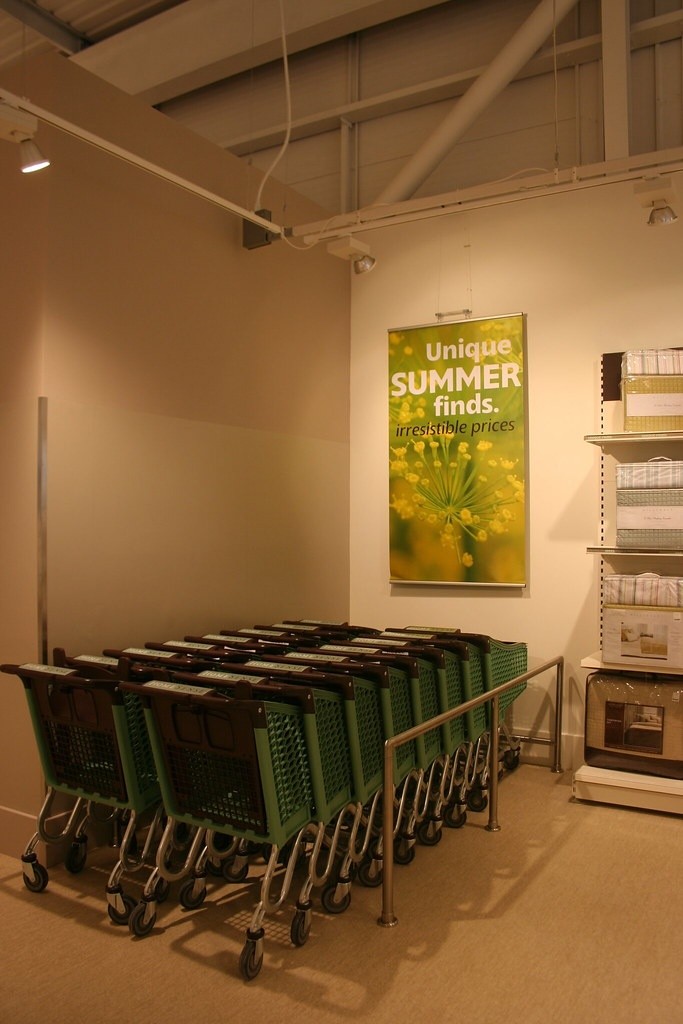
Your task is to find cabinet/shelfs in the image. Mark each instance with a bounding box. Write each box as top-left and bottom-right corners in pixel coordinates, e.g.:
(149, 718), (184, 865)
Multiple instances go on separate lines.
(573, 429), (683, 817)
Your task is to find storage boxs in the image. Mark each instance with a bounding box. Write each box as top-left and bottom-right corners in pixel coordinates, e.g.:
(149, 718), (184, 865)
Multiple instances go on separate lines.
(603, 607), (683, 676)
(615, 489), (683, 551)
(622, 376), (683, 430)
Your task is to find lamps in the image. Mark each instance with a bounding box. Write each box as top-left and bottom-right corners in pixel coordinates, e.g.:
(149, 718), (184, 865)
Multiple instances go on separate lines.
(349, 253), (377, 276)
(645, 200), (679, 227)
(13, 131), (50, 174)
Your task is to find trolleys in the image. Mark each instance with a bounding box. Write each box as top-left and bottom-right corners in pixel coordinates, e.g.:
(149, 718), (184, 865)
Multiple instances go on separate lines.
(0, 618), (527, 982)
